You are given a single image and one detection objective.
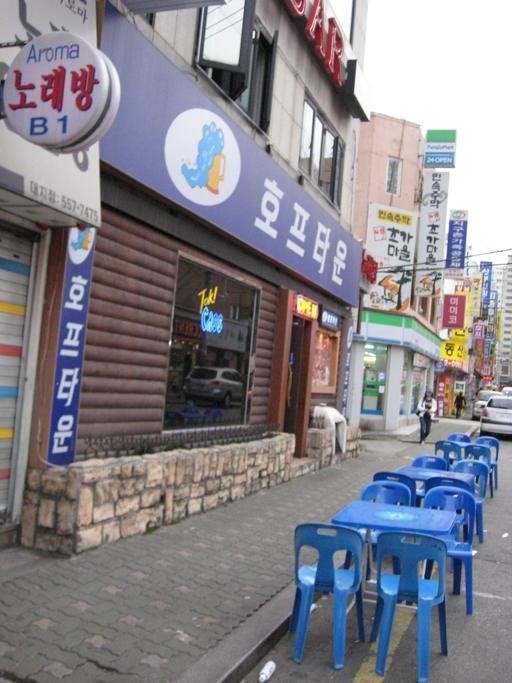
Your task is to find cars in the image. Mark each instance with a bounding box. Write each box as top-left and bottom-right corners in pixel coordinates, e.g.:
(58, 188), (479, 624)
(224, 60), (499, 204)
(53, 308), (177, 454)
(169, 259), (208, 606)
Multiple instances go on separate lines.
(471, 386), (512, 436)
(184, 365), (243, 409)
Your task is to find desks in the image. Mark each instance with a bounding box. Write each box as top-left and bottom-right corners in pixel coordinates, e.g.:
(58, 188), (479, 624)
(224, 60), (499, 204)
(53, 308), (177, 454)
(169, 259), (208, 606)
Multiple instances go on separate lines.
(332, 498), (457, 574)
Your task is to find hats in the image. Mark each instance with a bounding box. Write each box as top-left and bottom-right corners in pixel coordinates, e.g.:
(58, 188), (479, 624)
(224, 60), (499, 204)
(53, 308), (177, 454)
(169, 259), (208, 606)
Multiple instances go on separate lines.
(427, 388), (432, 392)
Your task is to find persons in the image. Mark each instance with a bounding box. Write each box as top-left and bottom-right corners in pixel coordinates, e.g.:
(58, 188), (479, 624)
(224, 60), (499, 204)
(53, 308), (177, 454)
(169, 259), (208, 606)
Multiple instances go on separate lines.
(416, 392), (437, 444)
(453, 392), (466, 419)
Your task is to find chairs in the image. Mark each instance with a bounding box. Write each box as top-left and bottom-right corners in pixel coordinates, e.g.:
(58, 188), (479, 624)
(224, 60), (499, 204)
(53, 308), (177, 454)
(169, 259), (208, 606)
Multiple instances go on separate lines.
(361, 480), (413, 555)
(422, 485), (476, 616)
(367, 531), (449, 683)
(373, 434), (497, 540)
(291, 522), (365, 671)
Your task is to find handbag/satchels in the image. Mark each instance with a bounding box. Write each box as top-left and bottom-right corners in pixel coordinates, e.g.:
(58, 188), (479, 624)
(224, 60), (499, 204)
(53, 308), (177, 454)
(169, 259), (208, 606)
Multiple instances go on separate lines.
(416, 410), (420, 416)
